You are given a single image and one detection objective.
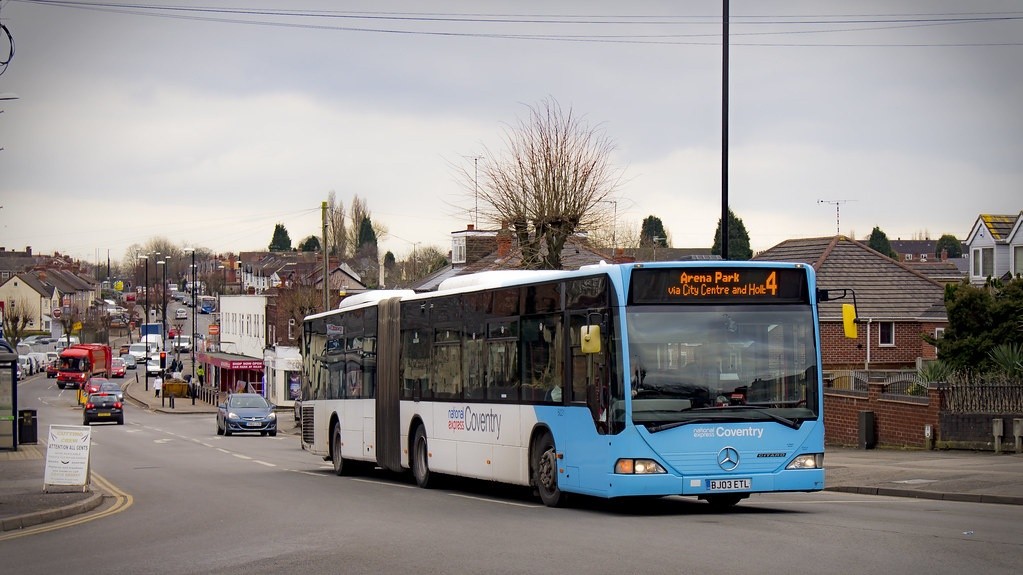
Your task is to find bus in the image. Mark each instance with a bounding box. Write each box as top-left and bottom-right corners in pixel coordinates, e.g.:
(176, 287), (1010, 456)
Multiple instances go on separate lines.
(298, 262), (861, 510)
(201, 296), (217, 314)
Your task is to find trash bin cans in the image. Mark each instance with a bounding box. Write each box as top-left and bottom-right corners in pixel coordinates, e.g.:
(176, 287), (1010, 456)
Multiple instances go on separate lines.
(857, 411), (875, 449)
(17, 408), (38, 445)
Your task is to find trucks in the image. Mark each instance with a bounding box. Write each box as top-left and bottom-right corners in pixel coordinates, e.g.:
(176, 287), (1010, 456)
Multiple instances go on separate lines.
(57, 344), (112, 389)
(54, 337), (81, 354)
(141, 323), (162, 338)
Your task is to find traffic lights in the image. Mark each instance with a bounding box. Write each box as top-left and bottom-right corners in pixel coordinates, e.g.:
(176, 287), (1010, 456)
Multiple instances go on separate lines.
(160, 352), (168, 370)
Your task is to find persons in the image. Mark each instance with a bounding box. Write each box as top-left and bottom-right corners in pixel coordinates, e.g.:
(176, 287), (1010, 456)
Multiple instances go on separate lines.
(614, 354), (648, 400)
(186, 375), (192, 398)
(165, 358), (184, 381)
(196, 365), (205, 387)
(551, 374), (563, 403)
(153, 375), (162, 398)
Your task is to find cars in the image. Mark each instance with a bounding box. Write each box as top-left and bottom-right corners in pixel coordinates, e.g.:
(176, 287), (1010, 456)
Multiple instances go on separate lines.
(98, 383), (125, 403)
(146, 356), (167, 377)
(18, 336), (59, 380)
(111, 360), (125, 378)
(171, 293), (178, 300)
(176, 293), (196, 308)
(83, 393), (125, 425)
(175, 308), (187, 320)
(216, 393), (278, 437)
(168, 327), (182, 339)
(84, 378), (108, 393)
(121, 354), (138, 369)
(126, 293), (136, 301)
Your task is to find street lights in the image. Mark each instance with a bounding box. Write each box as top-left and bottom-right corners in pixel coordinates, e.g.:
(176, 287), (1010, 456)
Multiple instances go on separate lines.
(153, 250), (172, 352)
(189, 265), (199, 355)
(184, 248), (197, 378)
(139, 255), (150, 390)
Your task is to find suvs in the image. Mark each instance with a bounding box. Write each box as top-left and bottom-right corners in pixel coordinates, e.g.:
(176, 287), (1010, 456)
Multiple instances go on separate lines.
(168, 284), (179, 293)
(120, 344), (131, 356)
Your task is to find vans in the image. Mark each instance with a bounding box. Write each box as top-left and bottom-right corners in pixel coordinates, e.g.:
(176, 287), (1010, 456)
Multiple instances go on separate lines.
(171, 335), (193, 353)
(141, 334), (163, 354)
(129, 344), (151, 363)
